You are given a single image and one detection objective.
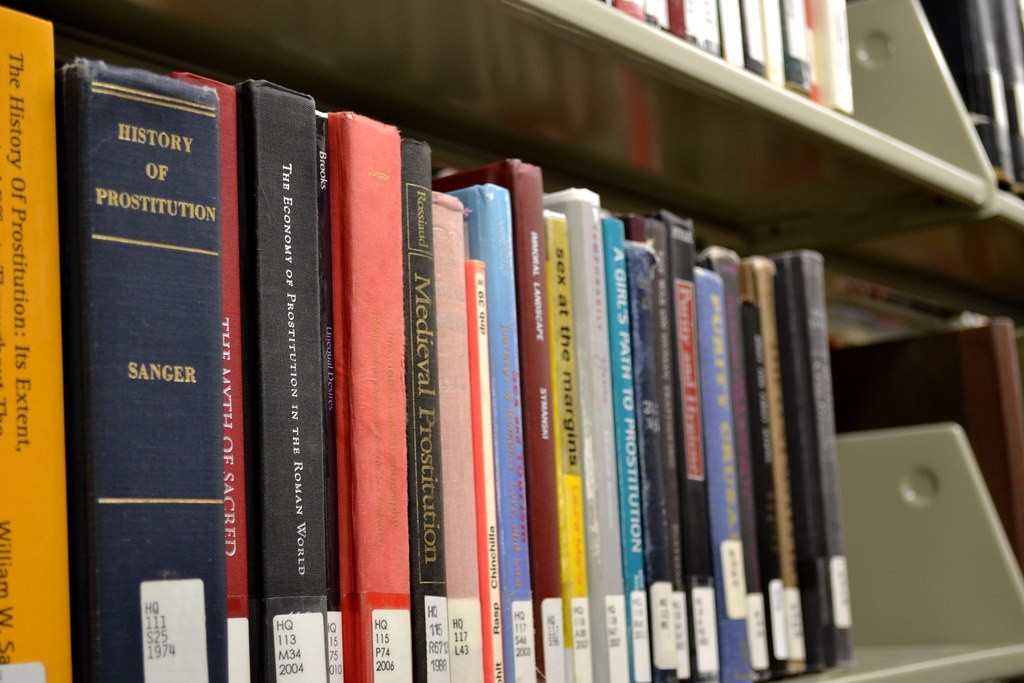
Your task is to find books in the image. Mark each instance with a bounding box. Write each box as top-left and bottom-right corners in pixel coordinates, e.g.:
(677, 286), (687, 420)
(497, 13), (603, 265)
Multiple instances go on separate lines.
(0, 1), (1024, 683)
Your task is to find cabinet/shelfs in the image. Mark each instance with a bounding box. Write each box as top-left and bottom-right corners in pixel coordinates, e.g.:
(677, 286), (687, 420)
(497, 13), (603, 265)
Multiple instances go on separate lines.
(36, 0), (1024, 683)
